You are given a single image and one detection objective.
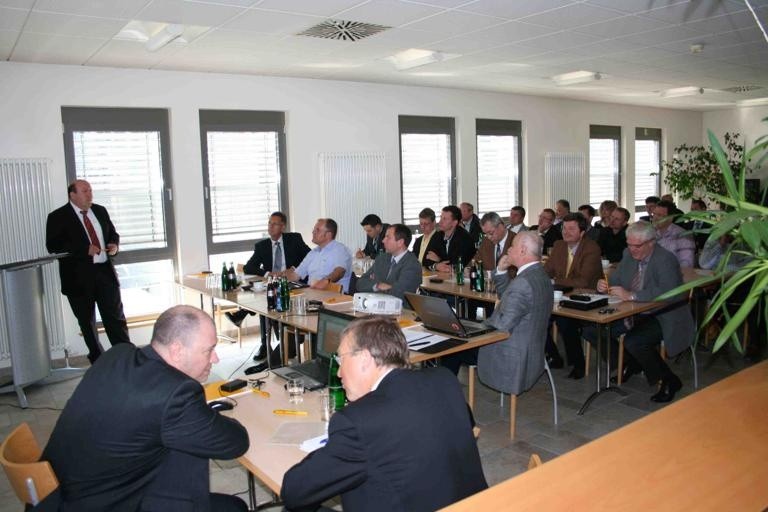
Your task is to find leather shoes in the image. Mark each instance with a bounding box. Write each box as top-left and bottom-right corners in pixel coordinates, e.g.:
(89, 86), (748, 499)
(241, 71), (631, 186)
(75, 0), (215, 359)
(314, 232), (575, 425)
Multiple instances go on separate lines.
(651, 375), (682, 402)
(609, 363), (641, 383)
(245, 344), (273, 375)
(548, 360), (585, 379)
(225, 312), (248, 326)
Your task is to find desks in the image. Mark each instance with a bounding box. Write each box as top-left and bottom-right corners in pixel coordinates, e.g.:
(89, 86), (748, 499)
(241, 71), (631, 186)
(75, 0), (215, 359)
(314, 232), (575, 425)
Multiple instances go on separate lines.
(437, 351), (768, 512)
(681, 266), (722, 289)
(175, 268), (510, 371)
(192, 363), (482, 512)
(422, 265), (666, 415)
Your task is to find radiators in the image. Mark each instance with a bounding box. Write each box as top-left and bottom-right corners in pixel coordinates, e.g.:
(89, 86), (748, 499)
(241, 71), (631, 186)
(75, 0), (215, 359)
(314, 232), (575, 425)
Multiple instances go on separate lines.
(542, 150), (588, 217)
(316, 148), (388, 261)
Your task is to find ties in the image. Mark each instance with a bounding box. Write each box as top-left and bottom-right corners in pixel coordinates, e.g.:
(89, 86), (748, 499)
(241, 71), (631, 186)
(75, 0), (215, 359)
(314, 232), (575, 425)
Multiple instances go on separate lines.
(273, 242), (282, 275)
(566, 251), (573, 278)
(624, 261), (645, 332)
(80, 211), (101, 255)
(496, 243), (500, 268)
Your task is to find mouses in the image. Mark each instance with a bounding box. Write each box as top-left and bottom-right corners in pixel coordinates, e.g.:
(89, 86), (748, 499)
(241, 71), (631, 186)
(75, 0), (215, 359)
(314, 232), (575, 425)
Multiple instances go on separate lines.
(210, 400), (234, 411)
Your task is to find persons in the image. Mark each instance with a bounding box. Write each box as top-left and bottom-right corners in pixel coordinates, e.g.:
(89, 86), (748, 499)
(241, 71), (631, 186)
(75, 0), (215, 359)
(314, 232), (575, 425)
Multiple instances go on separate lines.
(46, 180), (130, 365)
(24, 305), (249, 512)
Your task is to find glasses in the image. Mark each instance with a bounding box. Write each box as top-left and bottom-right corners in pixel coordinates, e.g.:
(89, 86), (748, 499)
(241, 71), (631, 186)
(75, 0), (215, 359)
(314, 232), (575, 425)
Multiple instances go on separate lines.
(333, 348), (363, 365)
(627, 239), (654, 248)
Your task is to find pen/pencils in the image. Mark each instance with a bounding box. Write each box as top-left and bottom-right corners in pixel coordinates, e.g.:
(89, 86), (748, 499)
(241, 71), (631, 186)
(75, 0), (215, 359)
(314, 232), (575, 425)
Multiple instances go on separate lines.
(604, 274), (609, 295)
(320, 438), (329, 443)
(253, 280), (262, 282)
(272, 410), (308, 415)
(359, 248), (363, 258)
(253, 389), (270, 396)
(409, 342), (430, 347)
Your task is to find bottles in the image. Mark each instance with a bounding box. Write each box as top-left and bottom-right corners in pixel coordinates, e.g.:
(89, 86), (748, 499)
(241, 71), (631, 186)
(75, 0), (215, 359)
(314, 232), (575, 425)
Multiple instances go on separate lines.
(379, 249), (383, 256)
(221, 261), (237, 291)
(266, 275), (289, 312)
(456, 256), (464, 285)
(329, 352), (346, 410)
(469, 260), (485, 291)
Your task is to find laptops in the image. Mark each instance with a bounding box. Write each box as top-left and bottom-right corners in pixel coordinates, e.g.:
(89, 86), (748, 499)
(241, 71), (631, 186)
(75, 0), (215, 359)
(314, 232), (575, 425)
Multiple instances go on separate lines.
(403, 292), (496, 339)
(271, 307), (357, 392)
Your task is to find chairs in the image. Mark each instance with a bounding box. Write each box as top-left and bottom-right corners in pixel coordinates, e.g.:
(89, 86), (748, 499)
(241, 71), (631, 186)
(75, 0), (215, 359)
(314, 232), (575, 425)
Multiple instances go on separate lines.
(0, 421), (64, 511)
(284, 320), (312, 365)
(618, 290), (698, 390)
(199, 271), (248, 349)
(553, 316), (599, 376)
(469, 293), (557, 440)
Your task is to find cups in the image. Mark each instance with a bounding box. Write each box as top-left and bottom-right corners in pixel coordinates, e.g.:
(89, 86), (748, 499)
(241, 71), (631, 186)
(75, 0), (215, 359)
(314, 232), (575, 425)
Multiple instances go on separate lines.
(287, 378), (305, 404)
(554, 290), (563, 300)
(601, 260), (609, 268)
(546, 248), (552, 255)
(317, 391), (336, 422)
(484, 271), (498, 298)
(205, 274), (222, 289)
(289, 295), (309, 313)
(365, 261), (372, 273)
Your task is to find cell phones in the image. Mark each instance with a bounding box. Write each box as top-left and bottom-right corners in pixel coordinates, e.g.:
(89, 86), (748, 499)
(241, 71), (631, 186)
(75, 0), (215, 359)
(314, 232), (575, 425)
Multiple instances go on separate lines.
(430, 277), (443, 283)
(220, 378), (247, 392)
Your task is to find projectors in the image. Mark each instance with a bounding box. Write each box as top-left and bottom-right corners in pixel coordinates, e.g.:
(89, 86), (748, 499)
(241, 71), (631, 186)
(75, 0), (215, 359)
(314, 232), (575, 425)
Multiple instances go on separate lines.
(353, 292), (404, 317)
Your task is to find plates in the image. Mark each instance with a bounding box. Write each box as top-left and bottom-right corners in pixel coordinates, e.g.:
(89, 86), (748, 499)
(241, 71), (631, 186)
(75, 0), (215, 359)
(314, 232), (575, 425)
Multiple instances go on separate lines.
(552, 296), (568, 302)
